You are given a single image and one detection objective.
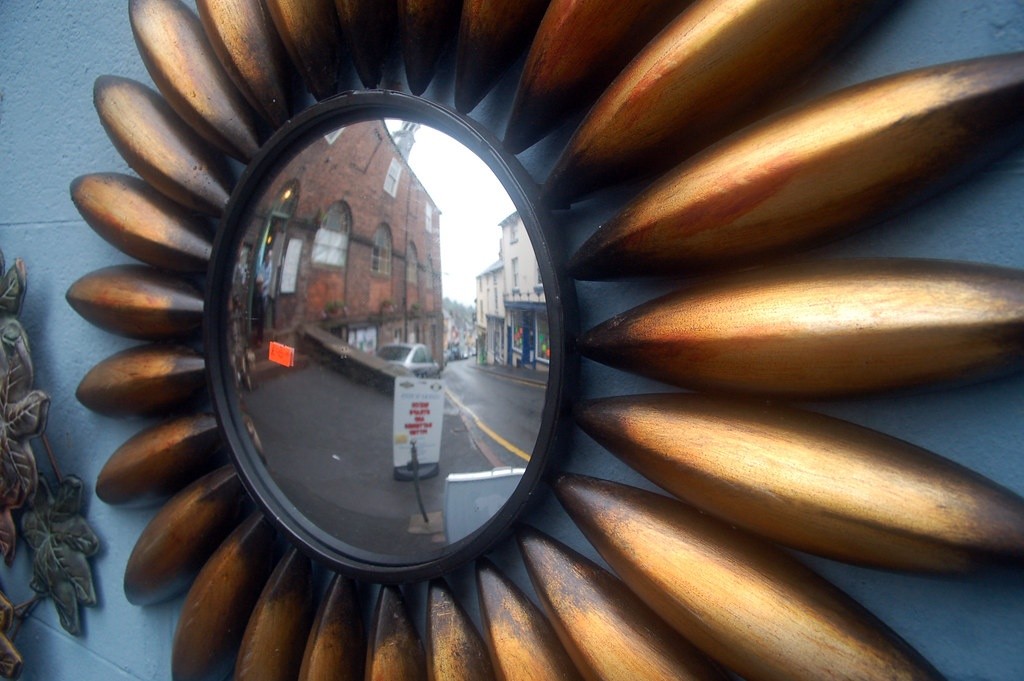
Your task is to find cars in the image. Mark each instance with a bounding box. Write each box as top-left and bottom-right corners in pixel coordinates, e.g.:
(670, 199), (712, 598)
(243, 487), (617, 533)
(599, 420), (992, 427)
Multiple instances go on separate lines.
(442, 345), (475, 367)
(377, 342), (441, 382)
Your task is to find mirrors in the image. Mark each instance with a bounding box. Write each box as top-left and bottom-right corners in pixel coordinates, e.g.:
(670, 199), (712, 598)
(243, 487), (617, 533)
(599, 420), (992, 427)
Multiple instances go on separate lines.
(202, 89), (581, 589)
